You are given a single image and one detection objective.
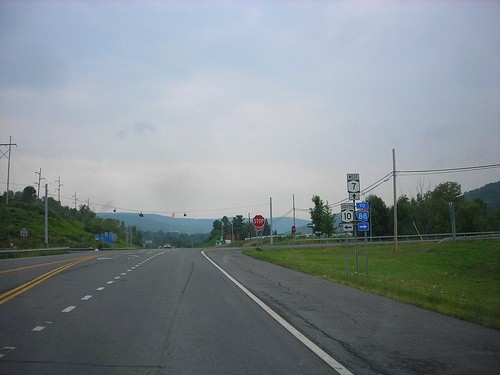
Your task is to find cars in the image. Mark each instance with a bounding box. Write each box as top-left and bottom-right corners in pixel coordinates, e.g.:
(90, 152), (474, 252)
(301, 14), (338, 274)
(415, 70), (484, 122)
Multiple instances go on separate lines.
(164, 243), (170, 248)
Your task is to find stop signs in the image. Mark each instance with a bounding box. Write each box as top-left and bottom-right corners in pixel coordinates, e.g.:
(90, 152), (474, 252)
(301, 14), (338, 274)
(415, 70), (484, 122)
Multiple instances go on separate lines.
(253, 215), (265, 227)
